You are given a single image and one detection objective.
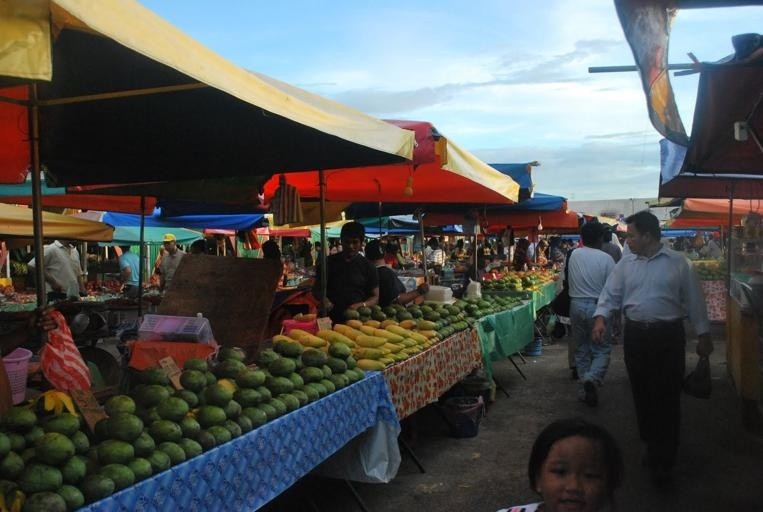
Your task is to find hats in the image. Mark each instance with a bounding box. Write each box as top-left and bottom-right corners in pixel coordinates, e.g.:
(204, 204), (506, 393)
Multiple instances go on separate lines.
(363, 240), (386, 260)
(162, 233), (178, 243)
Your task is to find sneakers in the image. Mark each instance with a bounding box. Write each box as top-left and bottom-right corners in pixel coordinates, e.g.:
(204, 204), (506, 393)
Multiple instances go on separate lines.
(583, 381), (600, 409)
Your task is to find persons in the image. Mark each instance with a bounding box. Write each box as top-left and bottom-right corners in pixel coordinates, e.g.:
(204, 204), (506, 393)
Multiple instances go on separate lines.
(397, 246), (405, 265)
(533, 239), (549, 267)
(385, 250), (398, 268)
(560, 316), (580, 380)
(311, 222), (380, 324)
(512, 238), (540, 271)
(156, 233), (187, 290)
(329, 245), (337, 255)
(566, 241), (575, 287)
(313, 241), (322, 279)
(425, 235), (509, 275)
(28, 239), (83, 304)
(554, 239), (568, 262)
(119, 246), (140, 288)
(560, 221), (617, 407)
(0, 303), (57, 414)
(497, 418), (624, 512)
(591, 211), (712, 493)
(336, 243), (343, 252)
(365, 240), (428, 306)
(600, 223), (621, 345)
(190, 240), (208, 255)
(262, 240), (280, 260)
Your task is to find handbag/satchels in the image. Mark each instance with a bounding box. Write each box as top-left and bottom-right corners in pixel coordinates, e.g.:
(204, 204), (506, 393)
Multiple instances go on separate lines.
(687, 355), (719, 401)
(550, 247), (575, 317)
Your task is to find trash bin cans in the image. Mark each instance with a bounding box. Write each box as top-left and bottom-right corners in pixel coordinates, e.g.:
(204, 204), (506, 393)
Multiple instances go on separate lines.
(1, 346), (32, 405)
(525, 335), (542, 357)
(445, 396), (486, 440)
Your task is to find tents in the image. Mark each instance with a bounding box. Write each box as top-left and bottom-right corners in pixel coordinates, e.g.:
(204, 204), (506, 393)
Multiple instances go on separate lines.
(658, 198), (763, 259)
(346, 162), (538, 287)
(0, 203), (113, 279)
(66, 120), (520, 316)
(61, 208), (311, 247)
(422, 192), (567, 282)
(1, 0), (413, 393)
(481, 212), (578, 262)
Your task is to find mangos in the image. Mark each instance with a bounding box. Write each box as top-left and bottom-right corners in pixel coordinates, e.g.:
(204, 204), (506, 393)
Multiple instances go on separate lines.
(272, 318), (440, 370)
(697, 264), (726, 281)
(454, 294), (526, 318)
(484, 272), (554, 291)
(0, 336), (367, 512)
(342, 304), (468, 340)
